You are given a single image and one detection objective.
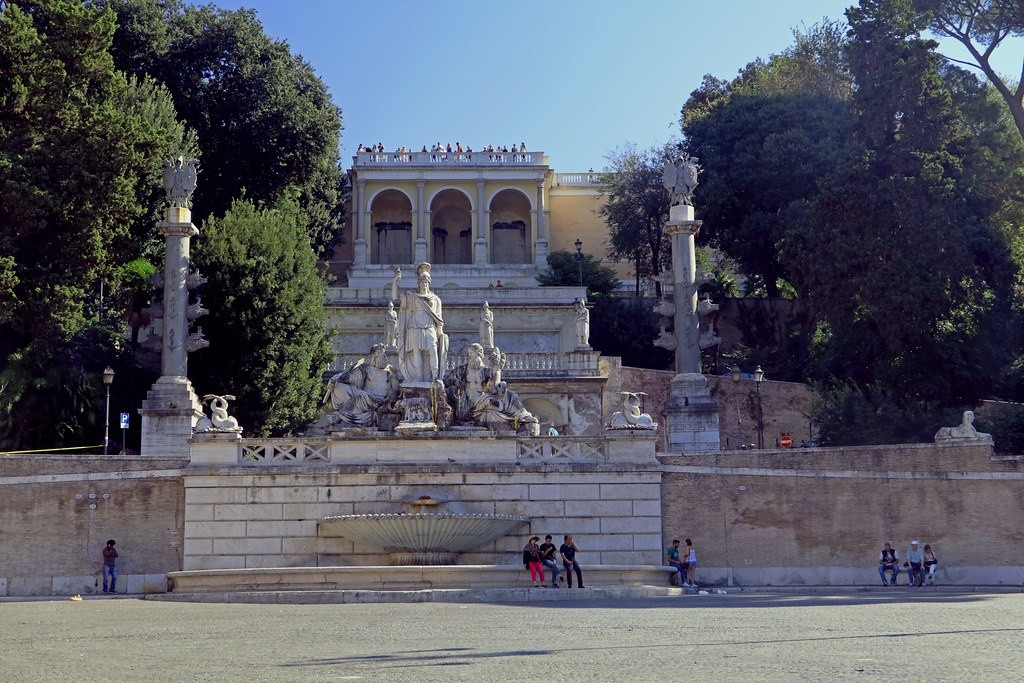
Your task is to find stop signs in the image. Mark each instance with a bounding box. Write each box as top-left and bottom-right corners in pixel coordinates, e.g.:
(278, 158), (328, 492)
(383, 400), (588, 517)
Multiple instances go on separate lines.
(781, 436), (792, 447)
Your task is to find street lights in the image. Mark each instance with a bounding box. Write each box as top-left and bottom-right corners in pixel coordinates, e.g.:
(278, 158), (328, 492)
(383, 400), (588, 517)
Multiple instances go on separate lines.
(102, 367), (115, 456)
(730, 363), (744, 450)
(754, 365), (765, 448)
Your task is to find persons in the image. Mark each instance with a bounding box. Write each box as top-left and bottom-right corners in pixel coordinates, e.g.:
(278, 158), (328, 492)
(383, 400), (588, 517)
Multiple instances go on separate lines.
(384, 301), (400, 348)
(560, 534), (586, 589)
(878, 542), (900, 587)
(326, 342), (401, 424)
(665, 539), (698, 588)
(450, 342), (538, 424)
(906, 541), (939, 587)
(539, 534), (564, 589)
(680, 538), (697, 588)
(479, 301), (495, 349)
(522, 536), (549, 589)
(574, 298), (590, 346)
(357, 142), (527, 163)
(392, 267), (445, 382)
(102, 539), (119, 596)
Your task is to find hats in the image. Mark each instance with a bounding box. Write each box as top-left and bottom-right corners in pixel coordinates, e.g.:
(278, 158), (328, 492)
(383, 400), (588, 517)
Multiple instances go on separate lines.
(910, 541), (919, 545)
(529, 535), (540, 543)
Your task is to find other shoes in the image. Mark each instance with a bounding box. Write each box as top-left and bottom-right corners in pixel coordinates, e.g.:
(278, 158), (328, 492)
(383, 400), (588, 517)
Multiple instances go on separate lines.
(910, 583), (912, 586)
(108, 591), (117, 595)
(890, 581), (897, 586)
(922, 583), (927, 586)
(534, 585), (538, 587)
(553, 583), (559, 588)
(542, 585), (548, 588)
(578, 586), (585, 588)
(681, 582), (691, 586)
(692, 583), (698, 587)
(560, 577), (565, 582)
(104, 591), (107, 594)
(568, 585), (572, 588)
(883, 580), (888, 585)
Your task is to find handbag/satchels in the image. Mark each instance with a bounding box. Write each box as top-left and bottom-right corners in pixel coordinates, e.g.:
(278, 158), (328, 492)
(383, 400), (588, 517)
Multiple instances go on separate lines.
(682, 558), (689, 569)
